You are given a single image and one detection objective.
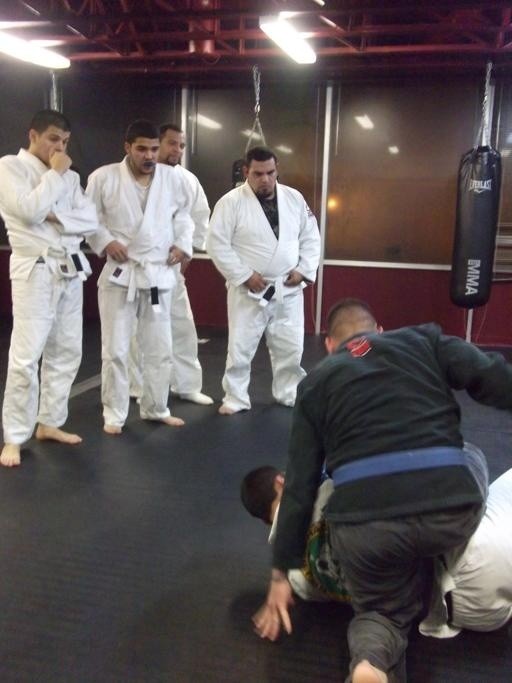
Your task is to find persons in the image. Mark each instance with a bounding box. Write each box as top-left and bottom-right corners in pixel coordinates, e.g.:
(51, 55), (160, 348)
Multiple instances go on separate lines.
(0, 109), (98, 467)
(252, 297), (512, 683)
(82, 120), (195, 436)
(126, 123), (214, 406)
(241, 464), (512, 640)
(204, 145), (321, 415)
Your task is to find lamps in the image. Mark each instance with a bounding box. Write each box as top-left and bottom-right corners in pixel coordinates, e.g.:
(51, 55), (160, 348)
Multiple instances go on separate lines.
(259, 14), (316, 65)
